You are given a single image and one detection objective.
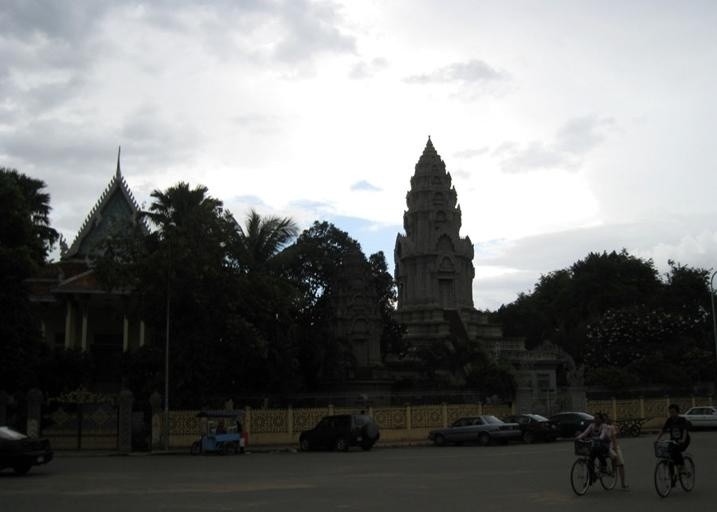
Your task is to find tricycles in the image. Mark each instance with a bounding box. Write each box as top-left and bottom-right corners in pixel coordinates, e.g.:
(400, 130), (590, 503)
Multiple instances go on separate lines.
(190, 409), (245, 457)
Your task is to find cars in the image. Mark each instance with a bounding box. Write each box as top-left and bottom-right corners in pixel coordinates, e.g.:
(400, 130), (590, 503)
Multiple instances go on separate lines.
(678, 407), (717, 430)
(546, 412), (595, 439)
(1, 426), (54, 475)
(429, 415), (520, 445)
(502, 413), (559, 444)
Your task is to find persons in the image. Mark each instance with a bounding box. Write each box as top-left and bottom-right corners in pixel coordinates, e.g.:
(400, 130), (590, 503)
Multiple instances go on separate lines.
(575, 411), (608, 487)
(600, 413), (630, 490)
(653, 402), (690, 490)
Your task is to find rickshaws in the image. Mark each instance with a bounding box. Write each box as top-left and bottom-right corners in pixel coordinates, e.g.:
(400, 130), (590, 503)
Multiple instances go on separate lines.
(609, 416), (654, 437)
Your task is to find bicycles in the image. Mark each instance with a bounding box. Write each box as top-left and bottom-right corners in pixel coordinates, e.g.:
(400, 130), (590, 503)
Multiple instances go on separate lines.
(570, 435), (618, 497)
(652, 439), (696, 498)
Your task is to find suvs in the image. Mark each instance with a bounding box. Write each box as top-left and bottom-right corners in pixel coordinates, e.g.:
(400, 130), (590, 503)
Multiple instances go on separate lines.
(300, 414), (380, 453)
